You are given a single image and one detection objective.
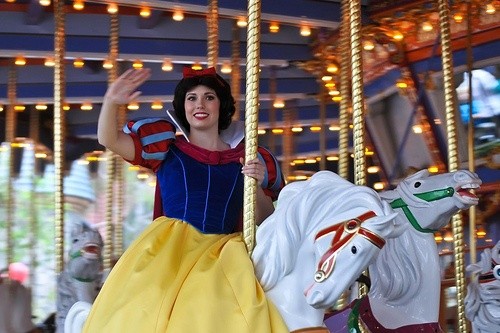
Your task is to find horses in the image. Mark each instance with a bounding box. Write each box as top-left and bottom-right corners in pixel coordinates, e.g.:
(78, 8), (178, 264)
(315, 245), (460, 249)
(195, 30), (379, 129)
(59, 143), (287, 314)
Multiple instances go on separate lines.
(464, 239), (500, 333)
(250, 170), (411, 333)
(55, 222), (112, 333)
(322, 168), (482, 332)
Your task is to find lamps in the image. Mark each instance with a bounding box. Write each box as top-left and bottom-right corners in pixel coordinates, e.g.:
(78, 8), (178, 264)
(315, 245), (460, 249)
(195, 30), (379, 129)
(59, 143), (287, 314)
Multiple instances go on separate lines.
(1, 0), (500, 242)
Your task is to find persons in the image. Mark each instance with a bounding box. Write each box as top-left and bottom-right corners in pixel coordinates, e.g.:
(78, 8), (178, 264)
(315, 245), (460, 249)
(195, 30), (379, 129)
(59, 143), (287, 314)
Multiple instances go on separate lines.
(81, 66), (291, 333)
